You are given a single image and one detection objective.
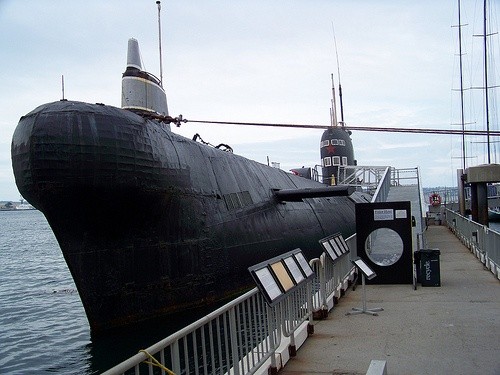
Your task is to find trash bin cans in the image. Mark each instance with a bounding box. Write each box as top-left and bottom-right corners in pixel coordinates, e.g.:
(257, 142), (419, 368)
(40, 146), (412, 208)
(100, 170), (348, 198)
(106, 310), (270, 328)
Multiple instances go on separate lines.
(413, 247), (442, 288)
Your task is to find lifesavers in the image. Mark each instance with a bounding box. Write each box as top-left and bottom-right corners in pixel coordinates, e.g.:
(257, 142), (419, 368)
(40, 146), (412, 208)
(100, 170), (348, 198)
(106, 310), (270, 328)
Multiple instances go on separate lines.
(429, 194), (442, 206)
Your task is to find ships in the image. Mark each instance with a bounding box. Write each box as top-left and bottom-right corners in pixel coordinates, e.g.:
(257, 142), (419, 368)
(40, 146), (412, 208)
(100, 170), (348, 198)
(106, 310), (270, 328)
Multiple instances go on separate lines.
(11, 0), (363, 343)
